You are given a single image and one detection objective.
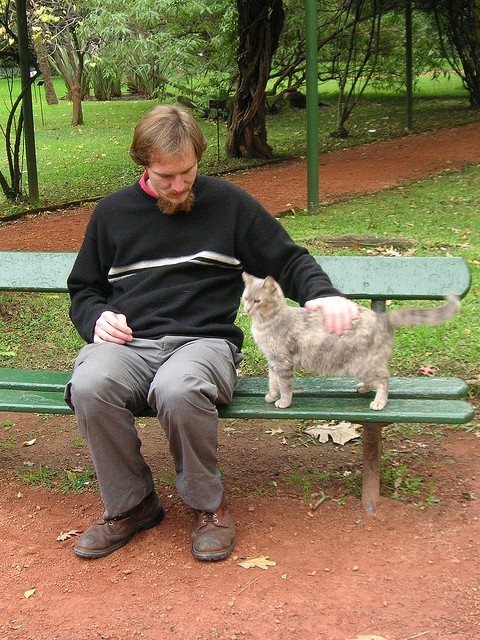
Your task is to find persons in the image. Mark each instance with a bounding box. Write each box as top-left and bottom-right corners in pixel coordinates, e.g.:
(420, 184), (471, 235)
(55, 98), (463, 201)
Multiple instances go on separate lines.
(63, 104), (359, 561)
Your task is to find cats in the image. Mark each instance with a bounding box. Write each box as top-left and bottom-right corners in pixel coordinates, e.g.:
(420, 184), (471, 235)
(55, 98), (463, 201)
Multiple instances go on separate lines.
(241, 270), (462, 411)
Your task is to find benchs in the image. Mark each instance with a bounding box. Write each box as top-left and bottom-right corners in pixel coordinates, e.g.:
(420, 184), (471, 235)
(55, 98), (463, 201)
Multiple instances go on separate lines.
(0, 251), (474, 512)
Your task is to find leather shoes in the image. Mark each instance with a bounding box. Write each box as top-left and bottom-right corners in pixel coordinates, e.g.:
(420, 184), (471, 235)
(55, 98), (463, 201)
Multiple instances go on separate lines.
(192, 492), (235, 562)
(74, 492), (164, 559)
